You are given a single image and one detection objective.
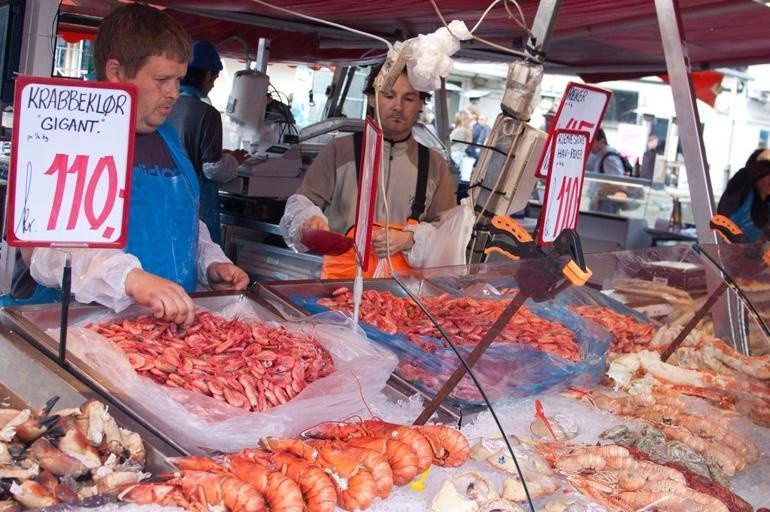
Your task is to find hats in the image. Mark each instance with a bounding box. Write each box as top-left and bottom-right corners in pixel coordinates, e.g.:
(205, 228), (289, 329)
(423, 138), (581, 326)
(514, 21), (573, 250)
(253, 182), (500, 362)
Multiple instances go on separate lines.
(187, 39), (222, 71)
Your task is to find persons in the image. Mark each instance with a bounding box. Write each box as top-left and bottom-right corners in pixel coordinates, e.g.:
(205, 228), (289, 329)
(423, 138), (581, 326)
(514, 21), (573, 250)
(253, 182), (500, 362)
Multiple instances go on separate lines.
(583, 126), (632, 218)
(715, 146), (770, 243)
(10, 3), (255, 326)
(633, 135), (659, 179)
(278, 61), (459, 282)
(422, 103), (495, 158)
(168, 35), (252, 243)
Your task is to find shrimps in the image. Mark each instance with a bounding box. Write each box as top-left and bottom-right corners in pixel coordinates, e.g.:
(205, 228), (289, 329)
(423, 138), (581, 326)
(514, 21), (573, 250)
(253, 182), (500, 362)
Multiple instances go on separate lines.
(83, 308), (340, 427)
(530, 392), (762, 512)
(314, 286), (657, 406)
(120, 417), (470, 512)
